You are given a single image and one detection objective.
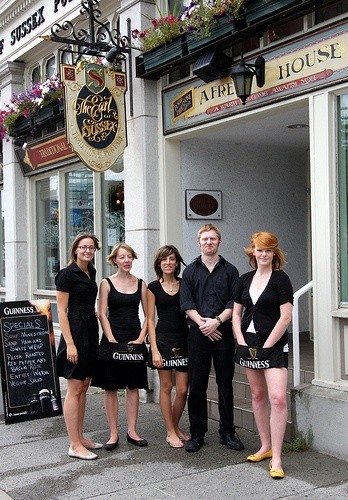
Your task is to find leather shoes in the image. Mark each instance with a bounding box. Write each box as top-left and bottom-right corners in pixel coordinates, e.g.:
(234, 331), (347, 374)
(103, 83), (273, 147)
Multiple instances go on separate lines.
(128, 434), (148, 447)
(104, 435), (119, 451)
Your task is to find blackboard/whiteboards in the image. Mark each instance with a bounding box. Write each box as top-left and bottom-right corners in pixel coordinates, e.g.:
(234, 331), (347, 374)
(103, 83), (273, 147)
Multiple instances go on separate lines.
(0, 300), (65, 424)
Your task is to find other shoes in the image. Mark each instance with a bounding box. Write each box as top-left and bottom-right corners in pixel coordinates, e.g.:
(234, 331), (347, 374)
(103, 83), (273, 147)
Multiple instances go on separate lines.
(247, 449), (285, 478)
(185, 435), (204, 452)
(68, 439), (103, 460)
(219, 432), (244, 450)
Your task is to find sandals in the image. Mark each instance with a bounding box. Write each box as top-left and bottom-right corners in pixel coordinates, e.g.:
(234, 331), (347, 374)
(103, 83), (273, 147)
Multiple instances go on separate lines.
(165, 429), (191, 448)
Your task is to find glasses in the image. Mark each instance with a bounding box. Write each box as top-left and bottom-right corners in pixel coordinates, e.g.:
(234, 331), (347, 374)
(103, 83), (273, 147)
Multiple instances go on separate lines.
(77, 245), (96, 252)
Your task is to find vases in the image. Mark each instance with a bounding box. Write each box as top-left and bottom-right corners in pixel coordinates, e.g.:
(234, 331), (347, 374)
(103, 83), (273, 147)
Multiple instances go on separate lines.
(185, 11), (240, 50)
(141, 38), (183, 73)
(58, 97), (65, 112)
(33, 104), (55, 123)
(11, 114), (33, 132)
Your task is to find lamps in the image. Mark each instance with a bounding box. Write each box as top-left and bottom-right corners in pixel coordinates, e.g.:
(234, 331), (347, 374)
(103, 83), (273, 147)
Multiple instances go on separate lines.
(227, 50), (265, 105)
(194, 50), (231, 83)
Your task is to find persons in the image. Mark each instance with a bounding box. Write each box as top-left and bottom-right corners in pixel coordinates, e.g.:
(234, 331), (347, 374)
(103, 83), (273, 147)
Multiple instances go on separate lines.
(97, 243), (150, 451)
(54, 234), (104, 460)
(179, 223), (244, 452)
(232, 231), (294, 478)
(145, 245), (191, 448)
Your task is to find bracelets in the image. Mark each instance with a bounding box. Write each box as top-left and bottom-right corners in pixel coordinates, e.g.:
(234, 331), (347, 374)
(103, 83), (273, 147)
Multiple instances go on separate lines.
(216, 316), (223, 324)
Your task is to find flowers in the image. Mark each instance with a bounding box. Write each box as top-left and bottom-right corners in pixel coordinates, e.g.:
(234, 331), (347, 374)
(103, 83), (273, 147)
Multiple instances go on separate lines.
(132, 0), (243, 53)
(0, 75), (65, 142)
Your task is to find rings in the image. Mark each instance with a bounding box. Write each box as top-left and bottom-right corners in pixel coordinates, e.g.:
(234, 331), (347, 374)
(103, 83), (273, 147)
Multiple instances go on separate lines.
(69, 358), (72, 359)
